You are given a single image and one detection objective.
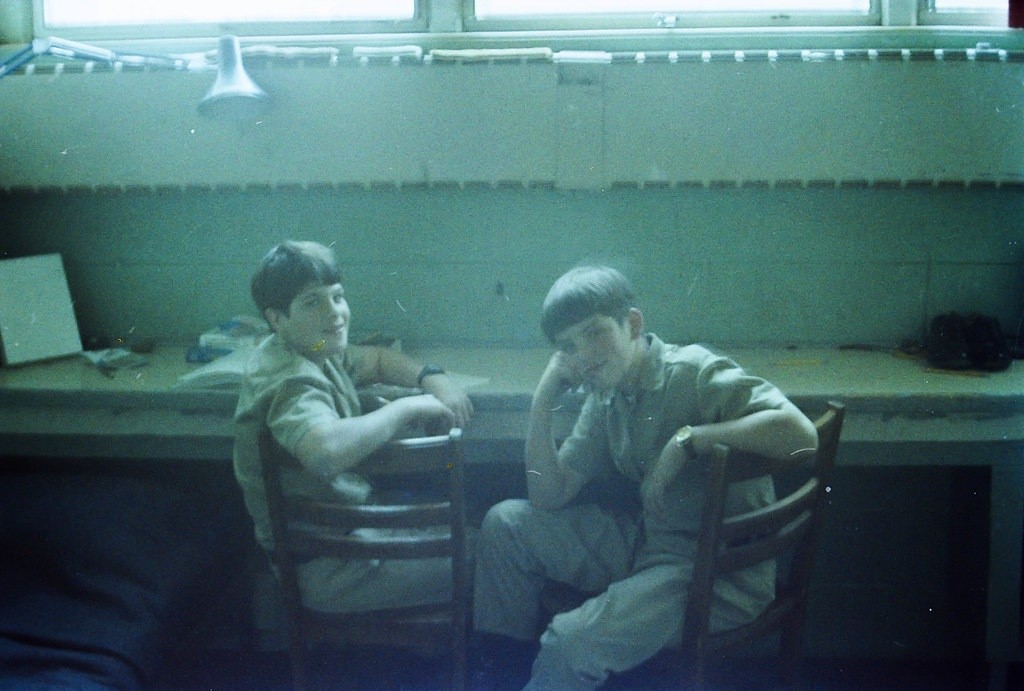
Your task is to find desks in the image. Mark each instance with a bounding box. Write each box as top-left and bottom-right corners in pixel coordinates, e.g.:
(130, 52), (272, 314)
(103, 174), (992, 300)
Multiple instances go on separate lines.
(0, 342), (1024, 691)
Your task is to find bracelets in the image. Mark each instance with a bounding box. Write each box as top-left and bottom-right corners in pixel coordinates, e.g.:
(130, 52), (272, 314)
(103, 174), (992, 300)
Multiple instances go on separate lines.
(416, 363), (446, 386)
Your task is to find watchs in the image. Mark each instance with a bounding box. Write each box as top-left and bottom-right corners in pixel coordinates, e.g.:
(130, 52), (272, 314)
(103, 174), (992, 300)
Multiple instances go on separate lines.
(677, 424), (700, 461)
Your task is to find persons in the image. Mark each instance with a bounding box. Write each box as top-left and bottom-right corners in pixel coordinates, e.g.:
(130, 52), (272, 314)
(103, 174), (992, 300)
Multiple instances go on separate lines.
(231, 238), (485, 625)
(466, 263), (817, 691)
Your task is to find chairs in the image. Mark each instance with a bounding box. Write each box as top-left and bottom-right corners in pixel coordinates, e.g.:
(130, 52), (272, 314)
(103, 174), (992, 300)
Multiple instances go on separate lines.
(542, 399), (847, 691)
(255, 428), (474, 691)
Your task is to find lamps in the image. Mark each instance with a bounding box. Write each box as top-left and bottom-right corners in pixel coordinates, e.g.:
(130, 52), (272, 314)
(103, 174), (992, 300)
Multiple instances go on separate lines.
(198, 36), (274, 124)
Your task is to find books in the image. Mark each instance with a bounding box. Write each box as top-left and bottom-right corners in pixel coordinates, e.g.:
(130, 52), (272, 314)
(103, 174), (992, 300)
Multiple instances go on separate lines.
(170, 334), (274, 390)
